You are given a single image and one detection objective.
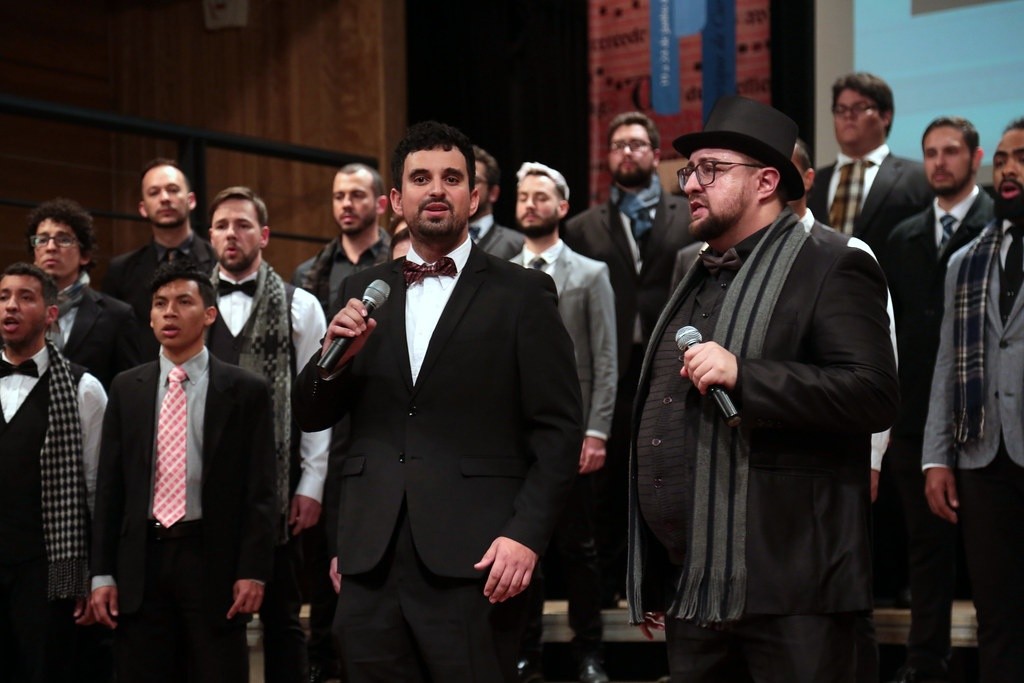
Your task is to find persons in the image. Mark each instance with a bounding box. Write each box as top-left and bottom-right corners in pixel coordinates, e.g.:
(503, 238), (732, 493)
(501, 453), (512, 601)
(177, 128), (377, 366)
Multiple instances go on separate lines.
(1, 73), (1024, 683)
(288, 120), (585, 683)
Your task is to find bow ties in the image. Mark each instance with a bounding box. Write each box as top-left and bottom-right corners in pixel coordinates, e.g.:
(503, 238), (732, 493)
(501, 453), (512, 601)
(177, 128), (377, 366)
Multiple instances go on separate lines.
(699, 247), (743, 276)
(0, 359), (40, 378)
(402, 257), (457, 285)
(218, 278), (257, 297)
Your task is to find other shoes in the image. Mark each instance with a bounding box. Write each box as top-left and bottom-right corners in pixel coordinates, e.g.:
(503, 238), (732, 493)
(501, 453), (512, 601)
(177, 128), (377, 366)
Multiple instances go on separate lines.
(306, 662), (338, 683)
(888, 658), (949, 683)
(578, 655), (609, 683)
(522, 656), (544, 683)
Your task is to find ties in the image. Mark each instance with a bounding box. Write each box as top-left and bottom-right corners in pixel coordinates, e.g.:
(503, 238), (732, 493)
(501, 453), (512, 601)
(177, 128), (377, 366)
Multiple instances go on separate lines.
(939, 215), (958, 258)
(168, 250), (177, 265)
(1004, 225), (1024, 316)
(468, 226), (480, 239)
(829, 159), (875, 237)
(152, 366), (189, 529)
(533, 258), (545, 268)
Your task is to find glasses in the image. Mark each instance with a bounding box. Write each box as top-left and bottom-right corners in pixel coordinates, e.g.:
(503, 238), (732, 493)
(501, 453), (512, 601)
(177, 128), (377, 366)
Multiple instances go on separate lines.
(29, 235), (77, 248)
(676, 160), (764, 191)
(834, 100), (877, 114)
(610, 140), (653, 152)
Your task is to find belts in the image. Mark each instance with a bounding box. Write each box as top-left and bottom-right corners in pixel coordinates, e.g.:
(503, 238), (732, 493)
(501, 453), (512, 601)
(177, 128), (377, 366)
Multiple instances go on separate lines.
(148, 521), (203, 540)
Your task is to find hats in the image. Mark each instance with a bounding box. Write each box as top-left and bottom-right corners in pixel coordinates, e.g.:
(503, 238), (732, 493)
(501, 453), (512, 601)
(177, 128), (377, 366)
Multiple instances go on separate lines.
(671, 94), (805, 201)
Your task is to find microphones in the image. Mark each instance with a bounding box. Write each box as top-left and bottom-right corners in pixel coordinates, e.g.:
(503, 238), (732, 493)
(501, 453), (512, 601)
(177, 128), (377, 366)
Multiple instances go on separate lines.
(676, 324), (742, 427)
(318, 280), (390, 371)
